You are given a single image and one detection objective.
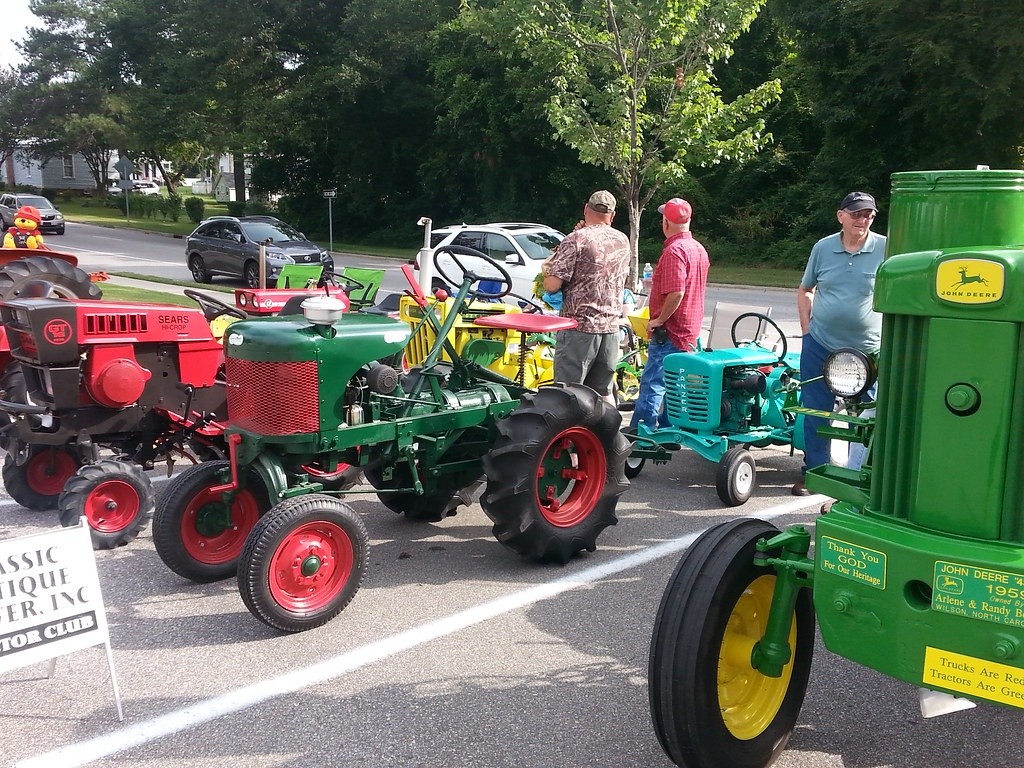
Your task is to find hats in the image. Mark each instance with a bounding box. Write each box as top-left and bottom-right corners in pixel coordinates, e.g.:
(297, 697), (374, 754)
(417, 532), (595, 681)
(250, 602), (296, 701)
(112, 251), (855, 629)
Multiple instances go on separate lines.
(587, 190), (617, 213)
(838, 192), (877, 212)
(658, 198), (692, 224)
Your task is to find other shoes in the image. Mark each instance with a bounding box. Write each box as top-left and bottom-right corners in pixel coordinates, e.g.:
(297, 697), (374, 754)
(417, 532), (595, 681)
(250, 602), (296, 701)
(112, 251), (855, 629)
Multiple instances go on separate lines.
(792, 475), (815, 496)
(660, 442), (682, 451)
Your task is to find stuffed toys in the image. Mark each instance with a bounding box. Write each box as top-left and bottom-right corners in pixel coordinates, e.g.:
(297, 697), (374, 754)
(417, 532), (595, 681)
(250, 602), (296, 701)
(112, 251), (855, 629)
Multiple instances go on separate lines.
(3, 206), (44, 249)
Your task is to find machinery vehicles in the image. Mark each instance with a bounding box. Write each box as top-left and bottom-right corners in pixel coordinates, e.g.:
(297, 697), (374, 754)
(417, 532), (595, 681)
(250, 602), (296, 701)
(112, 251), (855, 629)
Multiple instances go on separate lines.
(0, 237), (387, 551)
(153, 218), (672, 633)
(649, 166), (1024, 768)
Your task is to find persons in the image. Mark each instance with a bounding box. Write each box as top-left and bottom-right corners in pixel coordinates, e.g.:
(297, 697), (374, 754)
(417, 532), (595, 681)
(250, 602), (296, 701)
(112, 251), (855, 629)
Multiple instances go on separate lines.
(790, 192), (887, 496)
(541, 190), (631, 406)
(619, 198), (712, 451)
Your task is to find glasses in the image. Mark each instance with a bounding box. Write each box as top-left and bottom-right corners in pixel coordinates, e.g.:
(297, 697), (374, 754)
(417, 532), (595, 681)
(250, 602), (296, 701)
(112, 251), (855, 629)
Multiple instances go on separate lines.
(842, 209), (877, 220)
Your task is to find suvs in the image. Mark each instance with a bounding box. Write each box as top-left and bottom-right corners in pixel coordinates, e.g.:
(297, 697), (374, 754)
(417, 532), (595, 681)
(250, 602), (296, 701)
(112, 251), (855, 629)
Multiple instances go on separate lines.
(414, 223), (567, 309)
(107, 179), (161, 198)
(185, 216), (334, 289)
(0, 193), (65, 235)
